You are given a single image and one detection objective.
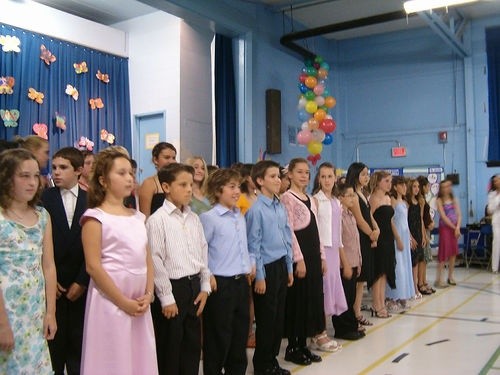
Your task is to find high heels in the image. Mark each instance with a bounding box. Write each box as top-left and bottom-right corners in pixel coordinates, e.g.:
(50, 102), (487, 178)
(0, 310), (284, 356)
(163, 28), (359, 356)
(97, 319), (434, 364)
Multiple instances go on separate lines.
(386, 300), (400, 311)
(370, 306), (392, 318)
(395, 299), (410, 309)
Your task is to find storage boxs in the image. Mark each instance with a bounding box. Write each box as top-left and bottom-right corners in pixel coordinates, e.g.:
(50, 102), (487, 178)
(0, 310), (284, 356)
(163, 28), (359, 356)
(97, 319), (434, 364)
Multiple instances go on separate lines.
(464, 230), (485, 258)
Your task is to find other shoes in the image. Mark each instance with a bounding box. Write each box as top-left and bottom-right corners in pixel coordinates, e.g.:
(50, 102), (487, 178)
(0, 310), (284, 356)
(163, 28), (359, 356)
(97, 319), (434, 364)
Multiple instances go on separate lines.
(434, 280), (447, 288)
(360, 302), (368, 311)
(414, 293), (422, 300)
(247, 335), (257, 347)
(337, 331), (366, 341)
(447, 277), (457, 285)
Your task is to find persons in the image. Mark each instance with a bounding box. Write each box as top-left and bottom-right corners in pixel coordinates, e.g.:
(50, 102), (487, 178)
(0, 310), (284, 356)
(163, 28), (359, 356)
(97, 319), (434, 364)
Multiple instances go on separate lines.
(97, 142), (257, 351)
(433, 179), (462, 288)
(146, 162), (212, 375)
(487, 174), (500, 275)
(246, 157), (438, 375)
(0, 148), (58, 375)
(198, 168), (253, 375)
(1, 135), (94, 375)
(79, 151), (160, 375)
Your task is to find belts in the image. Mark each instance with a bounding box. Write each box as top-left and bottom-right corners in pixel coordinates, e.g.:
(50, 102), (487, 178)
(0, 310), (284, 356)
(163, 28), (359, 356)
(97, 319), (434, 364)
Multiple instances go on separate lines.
(214, 275), (243, 279)
(169, 273), (199, 283)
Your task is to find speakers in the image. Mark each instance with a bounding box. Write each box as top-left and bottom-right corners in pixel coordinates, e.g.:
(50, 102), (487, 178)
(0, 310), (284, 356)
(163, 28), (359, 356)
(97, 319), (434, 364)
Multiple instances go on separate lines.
(266, 90), (281, 155)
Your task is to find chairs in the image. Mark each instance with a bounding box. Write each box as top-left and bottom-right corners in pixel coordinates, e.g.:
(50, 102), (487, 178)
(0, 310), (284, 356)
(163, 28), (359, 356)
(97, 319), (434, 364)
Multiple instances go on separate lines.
(429, 227), (439, 249)
(457, 227), (470, 268)
(470, 224), (492, 270)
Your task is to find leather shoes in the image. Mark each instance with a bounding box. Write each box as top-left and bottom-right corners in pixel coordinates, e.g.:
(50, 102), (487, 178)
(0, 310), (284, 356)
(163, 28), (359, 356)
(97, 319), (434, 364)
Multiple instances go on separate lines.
(298, 347), (321, 362)
(269, 365), (291, 375)
(285, 350), (311, 365)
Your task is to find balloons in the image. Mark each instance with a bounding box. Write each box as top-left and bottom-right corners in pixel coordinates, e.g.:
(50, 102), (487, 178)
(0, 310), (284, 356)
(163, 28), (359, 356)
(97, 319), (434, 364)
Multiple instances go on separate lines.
(296, 55), (336, 165)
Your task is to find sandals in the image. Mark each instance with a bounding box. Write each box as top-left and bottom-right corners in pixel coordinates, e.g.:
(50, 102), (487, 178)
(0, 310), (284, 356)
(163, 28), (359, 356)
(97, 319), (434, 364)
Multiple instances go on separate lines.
(417, 284), (432, 294)
(356, 315), (374, 325)
(323, 334), (342, 349)
(358, 324), (365, 332)
(309, 335), (338, 352)
(424, 283), (436, 293)
